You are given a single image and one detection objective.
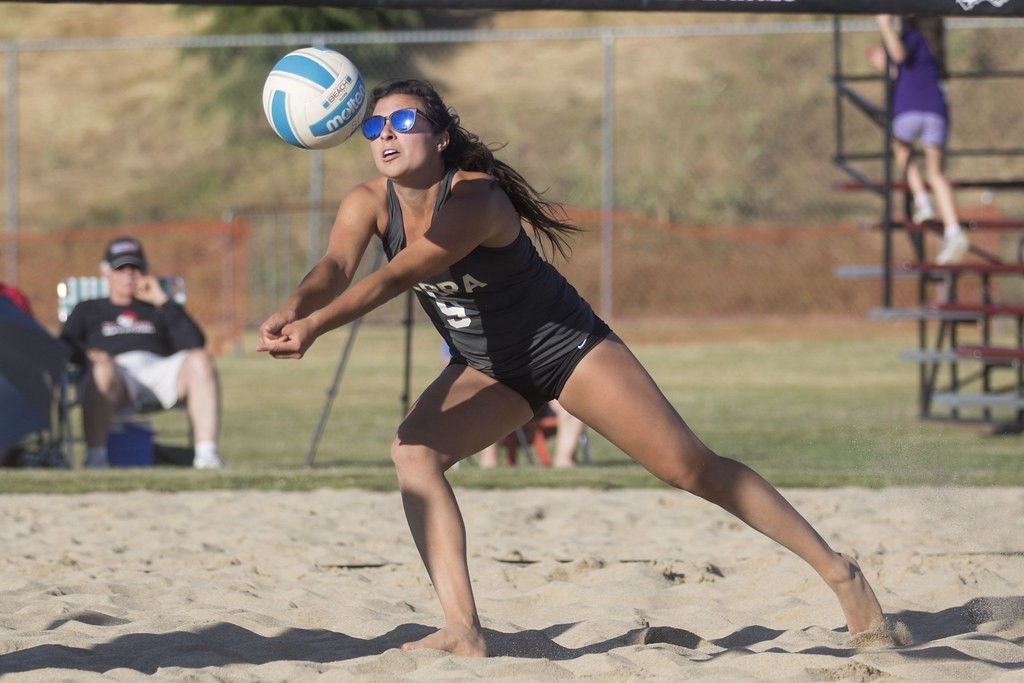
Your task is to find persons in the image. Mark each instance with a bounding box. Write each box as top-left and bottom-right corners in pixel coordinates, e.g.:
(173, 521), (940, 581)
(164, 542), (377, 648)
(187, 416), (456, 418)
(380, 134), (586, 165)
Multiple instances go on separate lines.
(864, 12), (972, 267)
(58, 236), (226, 469)
(440, 337), (586, 471)
(255, 78), (884, 658)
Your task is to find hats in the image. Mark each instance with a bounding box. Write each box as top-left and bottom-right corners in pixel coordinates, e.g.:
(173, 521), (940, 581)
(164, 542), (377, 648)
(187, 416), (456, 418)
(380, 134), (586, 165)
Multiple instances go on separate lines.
(106, 239), (145, 273)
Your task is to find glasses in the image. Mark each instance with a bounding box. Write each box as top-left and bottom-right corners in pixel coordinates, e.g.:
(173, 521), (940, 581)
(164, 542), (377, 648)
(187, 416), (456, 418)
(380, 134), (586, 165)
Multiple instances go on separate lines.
(360, 109), (445, 140)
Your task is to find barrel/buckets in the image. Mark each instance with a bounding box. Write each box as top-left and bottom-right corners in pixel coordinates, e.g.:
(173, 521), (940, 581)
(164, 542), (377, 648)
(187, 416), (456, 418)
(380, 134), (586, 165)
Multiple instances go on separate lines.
(108, 420), (155, 466)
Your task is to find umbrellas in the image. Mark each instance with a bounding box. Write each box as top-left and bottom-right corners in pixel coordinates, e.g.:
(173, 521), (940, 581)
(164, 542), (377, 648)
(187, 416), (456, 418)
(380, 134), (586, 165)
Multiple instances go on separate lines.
(0, 295), (74, 466)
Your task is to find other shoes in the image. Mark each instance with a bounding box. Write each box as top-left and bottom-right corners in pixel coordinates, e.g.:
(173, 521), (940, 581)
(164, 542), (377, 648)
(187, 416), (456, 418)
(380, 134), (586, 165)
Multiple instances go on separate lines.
(84, 456), (109, 469)
(193, 454), (223, 472)
(935, 234), (970, 267)
(912, 211), (935, 223)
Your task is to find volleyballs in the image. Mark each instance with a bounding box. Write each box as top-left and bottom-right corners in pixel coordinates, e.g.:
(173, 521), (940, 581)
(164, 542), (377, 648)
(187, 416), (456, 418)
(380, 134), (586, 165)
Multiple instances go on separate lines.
(261, 46), (368, 152)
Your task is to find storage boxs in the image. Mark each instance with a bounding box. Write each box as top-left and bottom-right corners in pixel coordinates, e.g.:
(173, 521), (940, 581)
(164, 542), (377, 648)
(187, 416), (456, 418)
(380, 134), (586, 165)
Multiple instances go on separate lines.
(106, 420), (157, 466)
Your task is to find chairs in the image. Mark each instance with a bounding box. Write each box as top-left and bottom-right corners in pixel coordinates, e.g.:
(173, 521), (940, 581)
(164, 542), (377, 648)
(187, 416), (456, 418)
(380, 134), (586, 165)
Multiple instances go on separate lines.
(55, 274), (195, 468)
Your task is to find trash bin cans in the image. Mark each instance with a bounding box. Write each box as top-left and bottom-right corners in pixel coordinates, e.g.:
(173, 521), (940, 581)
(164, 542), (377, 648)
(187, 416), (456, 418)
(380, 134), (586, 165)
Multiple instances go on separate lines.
(109, 421), (154, 465)
